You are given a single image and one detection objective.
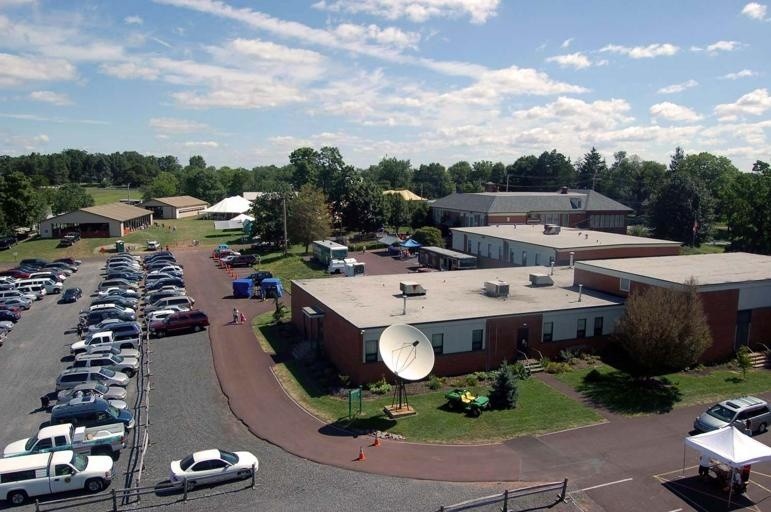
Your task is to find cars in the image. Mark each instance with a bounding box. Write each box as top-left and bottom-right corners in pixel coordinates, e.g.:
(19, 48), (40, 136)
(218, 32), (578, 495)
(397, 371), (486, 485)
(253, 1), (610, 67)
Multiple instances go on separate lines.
(0, 236), (18, 249)
(60, 230), (81, 246)
(147, 240), (160, 249)
(214, 244), (255, 267)
(694, 394), (771, 437)
(169, 448), (259, 491)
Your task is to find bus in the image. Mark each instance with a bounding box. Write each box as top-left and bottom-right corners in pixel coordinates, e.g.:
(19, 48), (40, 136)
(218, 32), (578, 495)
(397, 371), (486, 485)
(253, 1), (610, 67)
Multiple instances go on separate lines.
(311, 240), (348, 265)
(418, 246), (479, 269)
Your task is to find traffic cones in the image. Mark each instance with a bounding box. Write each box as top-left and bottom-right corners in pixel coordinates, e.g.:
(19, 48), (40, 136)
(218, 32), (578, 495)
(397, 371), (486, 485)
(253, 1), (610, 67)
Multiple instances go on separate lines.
(357, 446), (367, 461)
(226, 267), (238, 279)
(373, 433), (380, 447)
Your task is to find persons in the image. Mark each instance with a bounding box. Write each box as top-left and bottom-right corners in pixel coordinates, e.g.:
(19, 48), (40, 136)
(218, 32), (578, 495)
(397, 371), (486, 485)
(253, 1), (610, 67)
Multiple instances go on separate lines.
(240, 313), (246, 325)
(699, 453), (751, 494)
(233, 308), (239, 324)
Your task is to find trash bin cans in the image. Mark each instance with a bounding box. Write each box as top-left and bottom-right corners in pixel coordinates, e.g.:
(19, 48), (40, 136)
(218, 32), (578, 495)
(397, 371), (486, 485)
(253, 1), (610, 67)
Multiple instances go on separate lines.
(116, 240), (124, 252)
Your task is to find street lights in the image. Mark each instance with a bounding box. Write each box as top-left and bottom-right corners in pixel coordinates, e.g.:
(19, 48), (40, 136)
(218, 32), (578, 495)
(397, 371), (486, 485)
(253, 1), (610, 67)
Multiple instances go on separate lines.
(283, 191), (289, 258)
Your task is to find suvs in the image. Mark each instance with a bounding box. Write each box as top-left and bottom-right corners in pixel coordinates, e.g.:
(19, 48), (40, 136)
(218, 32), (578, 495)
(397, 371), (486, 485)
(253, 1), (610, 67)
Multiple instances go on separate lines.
(445, 388), (491, 417)
(247, 271), (273, 286)
